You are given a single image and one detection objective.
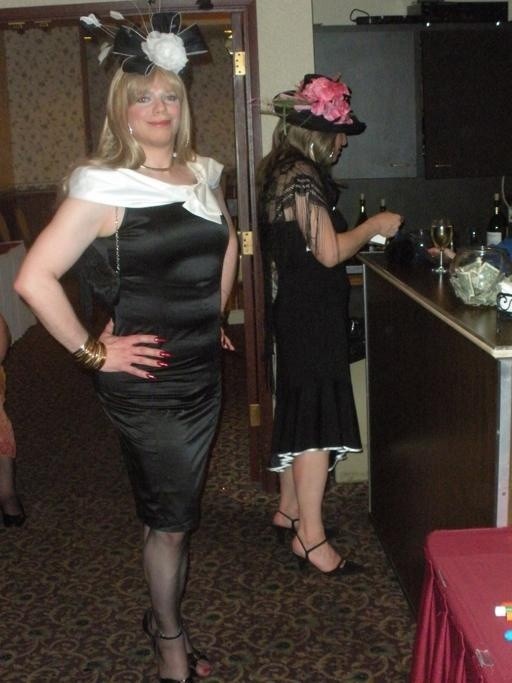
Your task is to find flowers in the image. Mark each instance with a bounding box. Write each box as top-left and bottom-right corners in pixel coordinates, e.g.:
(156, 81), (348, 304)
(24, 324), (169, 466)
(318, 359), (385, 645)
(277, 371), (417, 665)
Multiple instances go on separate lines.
(245, 75), (355, 133)
(140, 30), (189, 76)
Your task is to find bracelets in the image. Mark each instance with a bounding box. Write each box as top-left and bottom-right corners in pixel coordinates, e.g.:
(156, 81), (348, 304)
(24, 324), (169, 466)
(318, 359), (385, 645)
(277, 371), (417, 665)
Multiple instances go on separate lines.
(70, 334), (108, 371)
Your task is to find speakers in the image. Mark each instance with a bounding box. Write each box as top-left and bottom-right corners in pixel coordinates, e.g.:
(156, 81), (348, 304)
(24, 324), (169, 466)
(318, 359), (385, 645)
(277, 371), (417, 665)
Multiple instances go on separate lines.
(313, 22), (512, 181)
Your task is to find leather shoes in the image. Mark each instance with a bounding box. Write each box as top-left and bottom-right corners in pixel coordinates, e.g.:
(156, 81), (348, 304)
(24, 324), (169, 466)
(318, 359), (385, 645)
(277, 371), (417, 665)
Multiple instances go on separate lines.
(4, 501), (24, 528)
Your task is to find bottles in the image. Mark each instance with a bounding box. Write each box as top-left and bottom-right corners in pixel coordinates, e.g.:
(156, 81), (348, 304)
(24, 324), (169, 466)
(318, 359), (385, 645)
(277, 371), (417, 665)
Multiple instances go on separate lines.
(375, 197), (392, 251)
(353, 193), (373, 251)
(485, 191), (505, 249)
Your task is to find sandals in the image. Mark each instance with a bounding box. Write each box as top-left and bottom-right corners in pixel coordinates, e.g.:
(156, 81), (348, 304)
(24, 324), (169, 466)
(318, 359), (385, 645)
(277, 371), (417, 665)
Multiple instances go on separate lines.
(141, 609), (208, 682)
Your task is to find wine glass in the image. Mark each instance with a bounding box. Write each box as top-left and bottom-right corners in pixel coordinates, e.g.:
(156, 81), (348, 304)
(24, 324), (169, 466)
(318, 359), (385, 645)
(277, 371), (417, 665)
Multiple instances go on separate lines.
(430, 218), (454, 275)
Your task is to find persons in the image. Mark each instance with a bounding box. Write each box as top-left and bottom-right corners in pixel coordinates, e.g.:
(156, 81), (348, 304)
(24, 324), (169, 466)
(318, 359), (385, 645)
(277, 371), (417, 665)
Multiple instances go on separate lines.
(16, 11), (242, 682)
(0, 310), (26, 527)
(255, 74), (405, 575)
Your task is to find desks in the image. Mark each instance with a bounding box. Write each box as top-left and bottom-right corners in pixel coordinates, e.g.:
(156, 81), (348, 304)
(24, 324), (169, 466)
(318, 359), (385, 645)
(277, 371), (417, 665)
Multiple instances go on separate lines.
(422, 526), (512, 682)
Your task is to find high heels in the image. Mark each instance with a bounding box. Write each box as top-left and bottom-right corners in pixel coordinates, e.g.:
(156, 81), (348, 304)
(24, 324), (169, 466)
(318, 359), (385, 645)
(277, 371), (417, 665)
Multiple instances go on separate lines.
(273, 510), (338, 543)
(292, 532), (364, 576)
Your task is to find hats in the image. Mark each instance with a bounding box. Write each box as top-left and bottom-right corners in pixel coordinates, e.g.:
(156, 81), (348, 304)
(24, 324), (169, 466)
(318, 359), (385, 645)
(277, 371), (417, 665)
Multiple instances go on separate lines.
(273, 74), (366, 134)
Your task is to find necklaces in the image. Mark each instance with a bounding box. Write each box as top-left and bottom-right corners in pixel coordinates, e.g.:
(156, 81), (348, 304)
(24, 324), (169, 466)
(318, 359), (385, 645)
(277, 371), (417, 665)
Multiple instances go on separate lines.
(139, 162), (177, 171)
